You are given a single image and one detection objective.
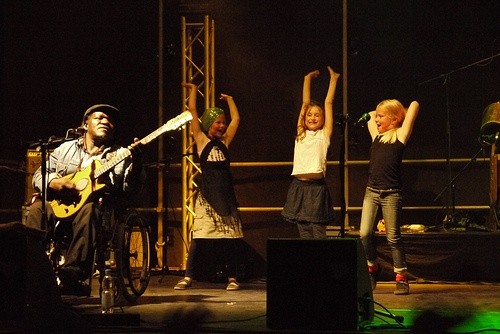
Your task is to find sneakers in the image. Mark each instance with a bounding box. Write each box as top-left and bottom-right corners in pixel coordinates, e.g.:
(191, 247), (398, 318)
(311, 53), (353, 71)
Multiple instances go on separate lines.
(394, 274), (409, 294)
(368, 266), (378, 291)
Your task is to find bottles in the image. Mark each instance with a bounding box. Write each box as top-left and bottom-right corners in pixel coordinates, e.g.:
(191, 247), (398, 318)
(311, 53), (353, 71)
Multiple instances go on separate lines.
(101, 269), (114, 314)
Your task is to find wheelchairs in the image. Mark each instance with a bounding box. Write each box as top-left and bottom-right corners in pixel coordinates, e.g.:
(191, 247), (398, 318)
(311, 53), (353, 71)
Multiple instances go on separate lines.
(21, 185), (151, 302)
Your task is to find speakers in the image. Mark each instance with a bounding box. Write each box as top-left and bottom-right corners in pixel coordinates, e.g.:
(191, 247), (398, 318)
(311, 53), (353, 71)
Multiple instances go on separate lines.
(185, 238), (265, 284)
(0, 221), (63, 320)
(265, 237), (374, 330)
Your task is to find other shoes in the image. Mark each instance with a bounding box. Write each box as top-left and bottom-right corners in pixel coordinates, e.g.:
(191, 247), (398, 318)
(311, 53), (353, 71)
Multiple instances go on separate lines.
(227, 279), (239, 291)
(59, 267), (82, 282)
(174, 279), (192, 289)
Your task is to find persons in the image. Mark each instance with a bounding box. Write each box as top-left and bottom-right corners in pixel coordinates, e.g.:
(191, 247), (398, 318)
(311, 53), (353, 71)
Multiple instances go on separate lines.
(359, 98), (420, 296)
(21, 102), (144, 297)
(174, 83), (245, 291)
(285, 66), (341, 238)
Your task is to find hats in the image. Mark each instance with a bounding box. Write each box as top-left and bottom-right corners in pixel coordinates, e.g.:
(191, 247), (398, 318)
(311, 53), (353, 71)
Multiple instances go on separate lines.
(83, 104), (120, 125)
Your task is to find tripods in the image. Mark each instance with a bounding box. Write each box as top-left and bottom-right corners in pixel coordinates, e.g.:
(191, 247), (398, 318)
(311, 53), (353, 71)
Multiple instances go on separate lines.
(418, 53), (500, 231)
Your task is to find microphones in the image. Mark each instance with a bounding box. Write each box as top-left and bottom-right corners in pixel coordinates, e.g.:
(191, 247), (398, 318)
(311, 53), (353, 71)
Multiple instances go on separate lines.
(73, 127), (88, 133)
(354, 113), (371, 129)
